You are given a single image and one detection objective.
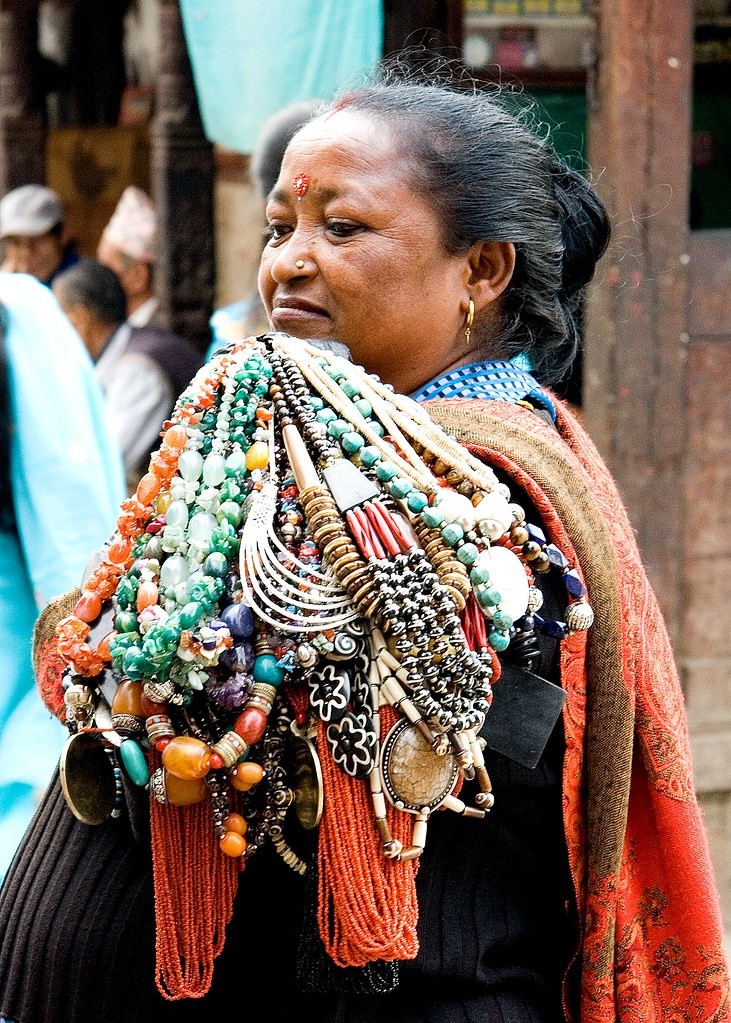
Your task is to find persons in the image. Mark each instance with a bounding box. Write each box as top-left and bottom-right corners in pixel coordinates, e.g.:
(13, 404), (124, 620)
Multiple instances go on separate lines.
(0, 84), (731, 1023)
(0, 180), (205, 492)
(205, 101), (337, 357)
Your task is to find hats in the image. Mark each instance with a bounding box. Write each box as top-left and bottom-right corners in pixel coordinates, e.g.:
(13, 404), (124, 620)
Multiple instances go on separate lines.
(0, 185), (61, 239)
(104, 186), (157, 263)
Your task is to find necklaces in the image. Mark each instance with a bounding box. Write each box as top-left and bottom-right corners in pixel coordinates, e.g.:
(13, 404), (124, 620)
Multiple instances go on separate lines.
(55, 333), (592, 996)
